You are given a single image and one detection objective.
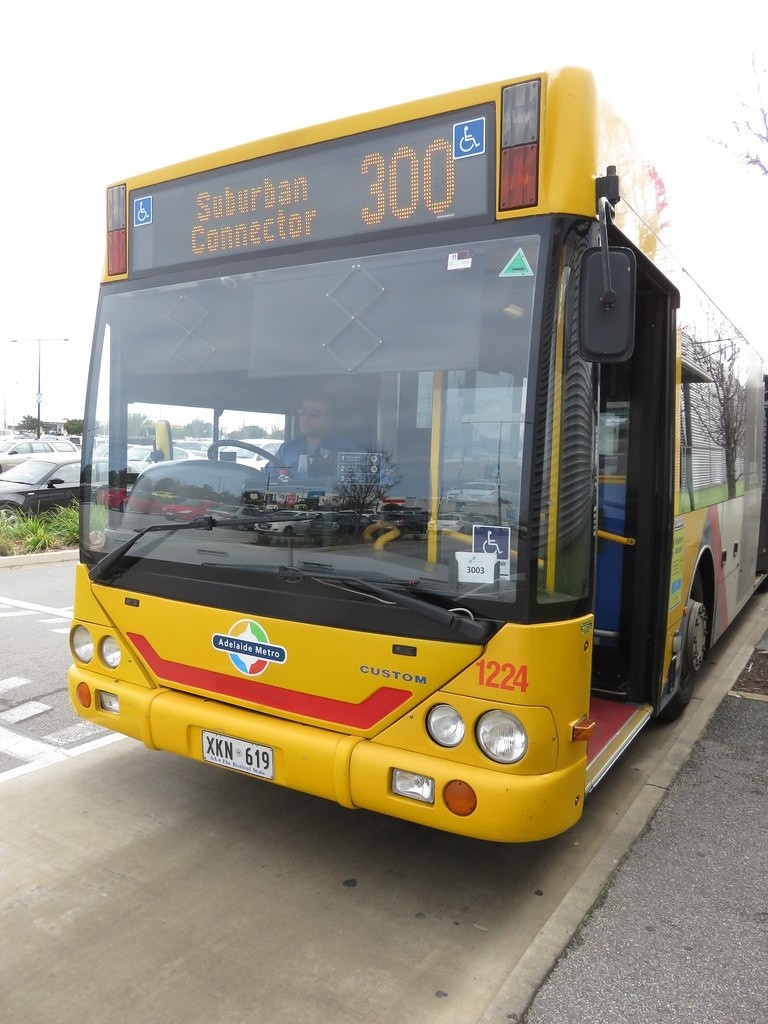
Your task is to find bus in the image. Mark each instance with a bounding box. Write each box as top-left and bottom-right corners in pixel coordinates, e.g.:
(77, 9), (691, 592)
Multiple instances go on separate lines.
(66, 65), (768, 848)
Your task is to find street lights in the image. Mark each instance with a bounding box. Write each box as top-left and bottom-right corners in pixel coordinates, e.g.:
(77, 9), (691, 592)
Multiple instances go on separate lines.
(12, 337), (69, 440)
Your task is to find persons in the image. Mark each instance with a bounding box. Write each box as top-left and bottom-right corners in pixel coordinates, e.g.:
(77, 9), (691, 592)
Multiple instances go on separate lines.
(261, 394), (367, 493)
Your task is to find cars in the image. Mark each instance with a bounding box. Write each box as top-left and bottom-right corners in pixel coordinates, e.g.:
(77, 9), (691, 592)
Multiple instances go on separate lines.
(0, 428), (286, 532)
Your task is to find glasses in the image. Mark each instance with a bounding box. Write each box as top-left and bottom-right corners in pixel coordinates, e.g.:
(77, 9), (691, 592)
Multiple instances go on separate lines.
(297, 408), (320, 417)
